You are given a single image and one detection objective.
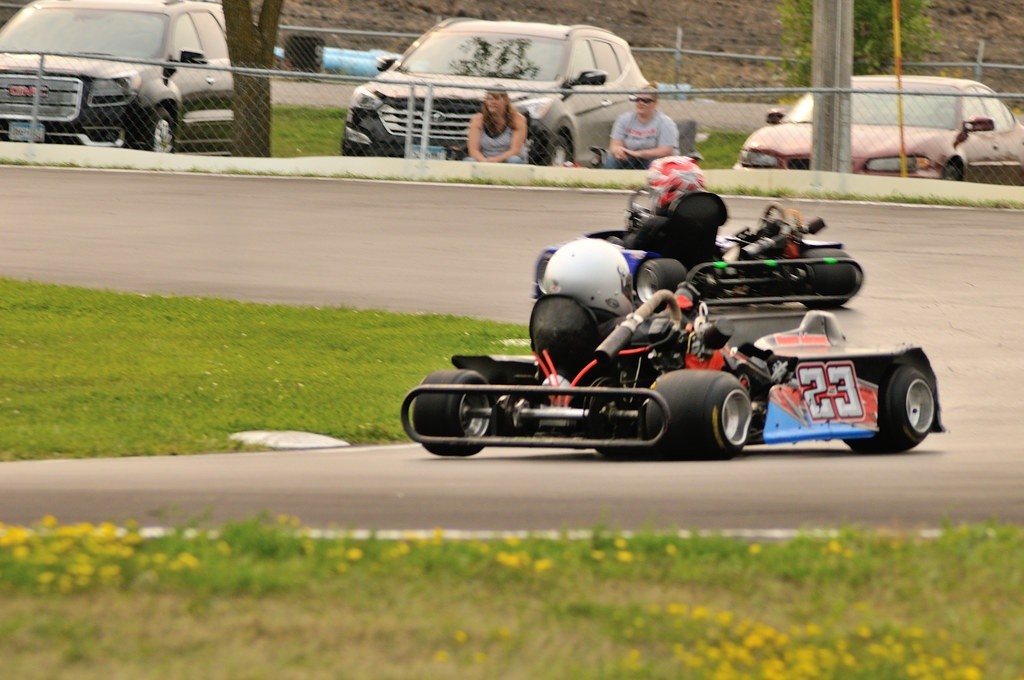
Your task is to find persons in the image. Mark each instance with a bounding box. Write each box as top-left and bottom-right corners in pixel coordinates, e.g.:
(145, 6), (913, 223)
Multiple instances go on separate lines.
(460, 83), (528, 165)
(543, 238), (695, 345)
(607, 154), (707, 246)
(608, 81), (679, 170)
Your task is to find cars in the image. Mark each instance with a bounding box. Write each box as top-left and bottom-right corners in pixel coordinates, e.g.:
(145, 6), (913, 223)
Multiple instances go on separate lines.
(341, 20), (637, 174)
(730, 71), (1024, 185)
(0, 0), (238, 156)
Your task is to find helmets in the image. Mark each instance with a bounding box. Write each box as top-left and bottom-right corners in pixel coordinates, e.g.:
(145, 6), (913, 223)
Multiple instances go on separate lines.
(646, 154), (706, 207)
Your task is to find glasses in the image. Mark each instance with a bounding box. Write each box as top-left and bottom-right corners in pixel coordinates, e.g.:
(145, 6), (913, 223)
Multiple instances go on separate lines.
(634, 95), (655, 103)
